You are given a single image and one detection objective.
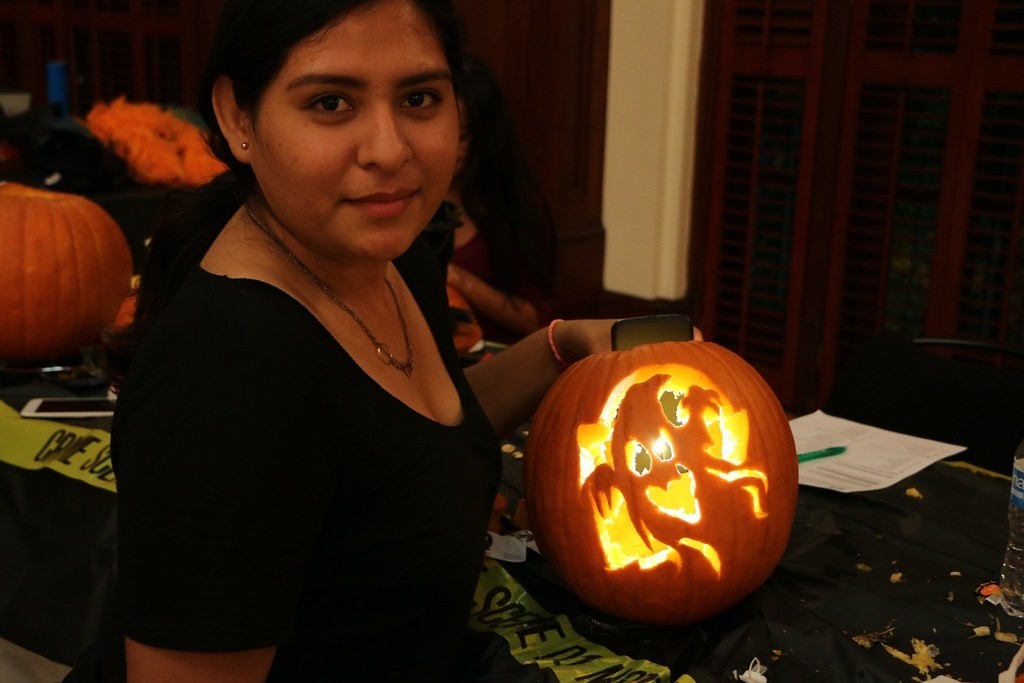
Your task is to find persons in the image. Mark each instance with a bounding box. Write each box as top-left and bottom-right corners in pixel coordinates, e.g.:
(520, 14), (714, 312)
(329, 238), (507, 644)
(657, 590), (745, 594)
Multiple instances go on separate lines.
(443, 45), (557, 365)
(62, 0), (704, 683)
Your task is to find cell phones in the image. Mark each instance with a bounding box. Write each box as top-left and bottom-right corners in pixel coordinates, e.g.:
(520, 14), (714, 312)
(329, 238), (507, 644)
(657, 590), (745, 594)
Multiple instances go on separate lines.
(20, 398), (117, 420)
(610, 314), (695, 352)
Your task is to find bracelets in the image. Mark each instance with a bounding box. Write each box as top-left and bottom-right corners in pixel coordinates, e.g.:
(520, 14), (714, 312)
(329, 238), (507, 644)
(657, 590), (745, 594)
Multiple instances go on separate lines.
(547, 318), (566, 367)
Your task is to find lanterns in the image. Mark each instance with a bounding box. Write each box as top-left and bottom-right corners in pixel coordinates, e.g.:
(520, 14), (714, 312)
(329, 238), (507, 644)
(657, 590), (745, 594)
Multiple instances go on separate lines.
(526, 339), (798, 625)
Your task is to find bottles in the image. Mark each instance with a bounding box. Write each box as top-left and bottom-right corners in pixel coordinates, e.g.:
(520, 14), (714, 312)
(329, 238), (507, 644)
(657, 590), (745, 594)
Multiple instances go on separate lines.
(998, 446), (1024, 618)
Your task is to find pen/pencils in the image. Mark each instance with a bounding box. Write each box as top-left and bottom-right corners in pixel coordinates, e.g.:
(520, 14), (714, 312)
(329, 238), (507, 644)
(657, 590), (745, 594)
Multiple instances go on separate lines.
(795, 447), (846, 463)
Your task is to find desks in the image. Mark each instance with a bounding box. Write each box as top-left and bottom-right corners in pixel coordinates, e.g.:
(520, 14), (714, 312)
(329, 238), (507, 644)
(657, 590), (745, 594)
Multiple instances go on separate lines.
(0, 339), (1023, 683)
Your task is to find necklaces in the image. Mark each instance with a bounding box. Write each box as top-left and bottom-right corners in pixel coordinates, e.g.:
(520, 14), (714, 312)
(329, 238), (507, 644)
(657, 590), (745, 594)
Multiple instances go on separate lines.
(243, 202), (416, 378)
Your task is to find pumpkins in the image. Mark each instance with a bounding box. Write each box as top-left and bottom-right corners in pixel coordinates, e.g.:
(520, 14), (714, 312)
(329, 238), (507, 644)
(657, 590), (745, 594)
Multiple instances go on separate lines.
(0, 182), (134, 361)
